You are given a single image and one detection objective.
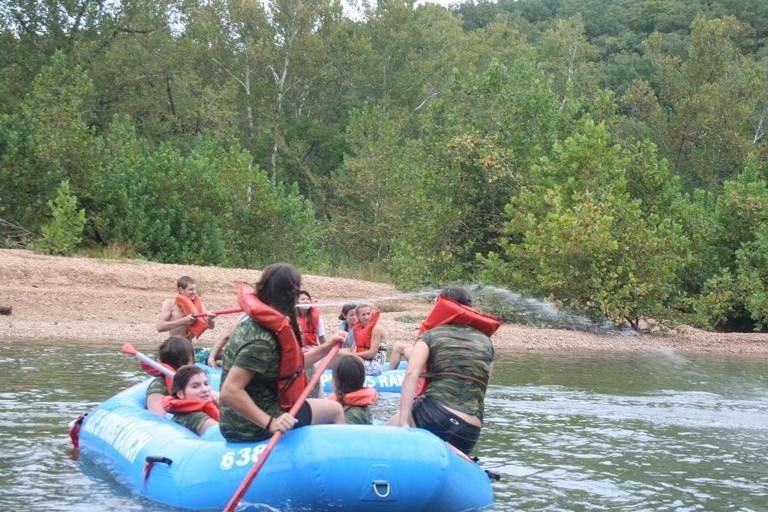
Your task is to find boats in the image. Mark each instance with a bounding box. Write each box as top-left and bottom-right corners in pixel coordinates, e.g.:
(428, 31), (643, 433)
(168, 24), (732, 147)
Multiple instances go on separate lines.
(185, 345), (408, 396)
(68, 368), (496, 512)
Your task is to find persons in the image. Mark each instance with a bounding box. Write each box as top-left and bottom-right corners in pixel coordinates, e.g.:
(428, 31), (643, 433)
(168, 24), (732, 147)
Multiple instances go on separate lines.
(389, 340), (414, 375)
(141, 263), (386, 444)
(389, 285), (495, 458)
